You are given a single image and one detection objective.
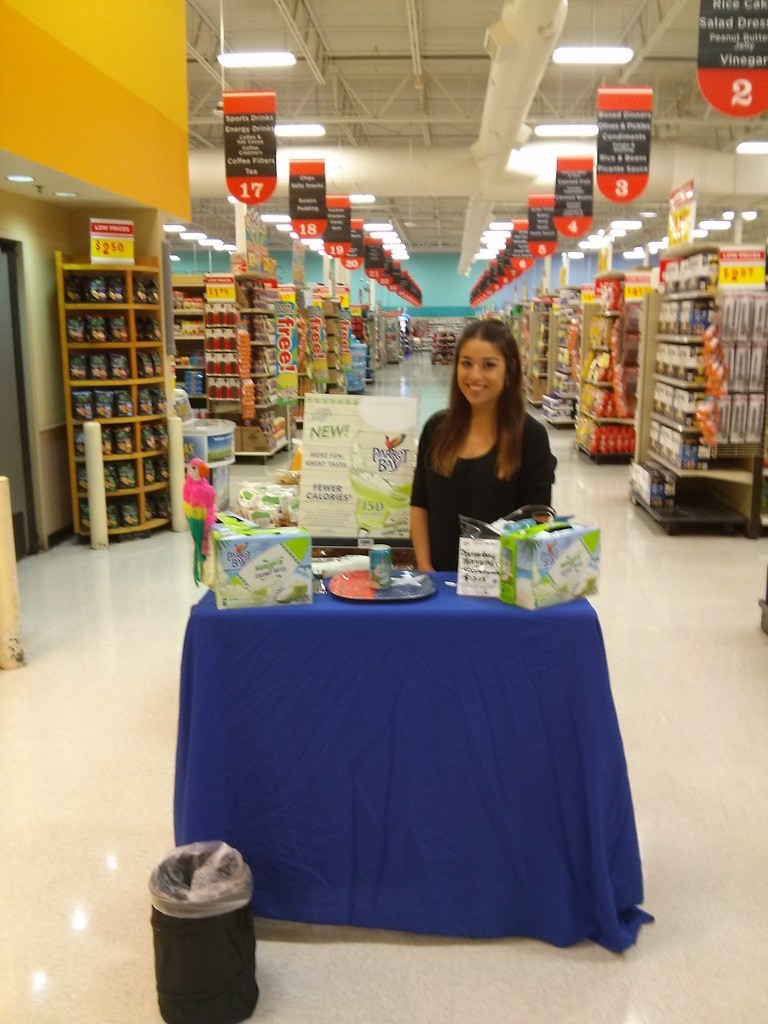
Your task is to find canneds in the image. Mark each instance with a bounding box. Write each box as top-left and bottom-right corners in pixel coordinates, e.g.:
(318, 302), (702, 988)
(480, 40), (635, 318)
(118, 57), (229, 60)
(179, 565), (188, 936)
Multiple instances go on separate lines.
(369, 544), (392, 590)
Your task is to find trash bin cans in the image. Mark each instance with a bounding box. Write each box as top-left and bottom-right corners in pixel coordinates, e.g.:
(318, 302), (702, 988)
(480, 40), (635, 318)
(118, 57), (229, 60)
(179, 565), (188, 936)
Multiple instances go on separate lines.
(147, 841), (260, 1024)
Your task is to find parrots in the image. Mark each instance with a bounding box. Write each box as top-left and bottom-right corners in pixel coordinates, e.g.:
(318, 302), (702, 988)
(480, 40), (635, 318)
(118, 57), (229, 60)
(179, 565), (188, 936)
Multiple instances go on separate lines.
(182, 457), (217, 588)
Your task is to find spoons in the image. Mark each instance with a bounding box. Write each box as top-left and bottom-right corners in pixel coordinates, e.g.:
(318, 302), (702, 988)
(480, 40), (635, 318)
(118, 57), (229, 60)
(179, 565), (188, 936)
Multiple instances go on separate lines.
(716, 300), (768, 439)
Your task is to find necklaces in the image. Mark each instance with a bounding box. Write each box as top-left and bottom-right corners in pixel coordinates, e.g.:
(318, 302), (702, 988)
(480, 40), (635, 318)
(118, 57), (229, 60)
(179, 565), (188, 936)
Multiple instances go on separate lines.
(469, 416), (496, 430)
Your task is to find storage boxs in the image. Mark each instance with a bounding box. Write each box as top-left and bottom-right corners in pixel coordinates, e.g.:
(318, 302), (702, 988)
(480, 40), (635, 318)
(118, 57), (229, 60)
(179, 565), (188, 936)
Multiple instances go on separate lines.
(212, 526), (313, 610)
(326, 319), (338, 333)
(499, 520), (602, 611)
(242, 426), (269, 452)
(195, 510), (260, 593)
(323, 302), (339, 314)
(327, 353), (339, 367)
(328, 337), (336, 351)
(328, 369), (339, 382)
(235, 427), (243, 452)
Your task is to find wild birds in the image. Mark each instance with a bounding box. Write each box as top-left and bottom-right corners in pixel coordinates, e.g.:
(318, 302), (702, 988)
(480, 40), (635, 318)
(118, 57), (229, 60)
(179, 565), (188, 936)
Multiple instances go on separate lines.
(385, 433), (406, 452)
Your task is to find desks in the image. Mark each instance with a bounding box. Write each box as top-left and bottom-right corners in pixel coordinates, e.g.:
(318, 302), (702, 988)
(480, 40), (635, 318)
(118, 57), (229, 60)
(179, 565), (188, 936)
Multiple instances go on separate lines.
(172, 567), (656, 955)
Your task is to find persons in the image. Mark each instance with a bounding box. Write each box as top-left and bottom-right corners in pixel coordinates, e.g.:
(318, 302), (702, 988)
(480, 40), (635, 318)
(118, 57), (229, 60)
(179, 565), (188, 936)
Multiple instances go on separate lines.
(410, 318), (558, 575)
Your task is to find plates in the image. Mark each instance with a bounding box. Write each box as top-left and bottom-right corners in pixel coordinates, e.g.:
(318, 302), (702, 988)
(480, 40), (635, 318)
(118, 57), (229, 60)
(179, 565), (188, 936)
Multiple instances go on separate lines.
(329, 570), (437, 599)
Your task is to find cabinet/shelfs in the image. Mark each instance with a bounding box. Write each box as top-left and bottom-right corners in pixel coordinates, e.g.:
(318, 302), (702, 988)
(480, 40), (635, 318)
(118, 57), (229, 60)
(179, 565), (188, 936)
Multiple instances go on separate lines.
(54, 250), (173, 546)
(502, 243), (768, 539)
(172, 271), (468, 465)
